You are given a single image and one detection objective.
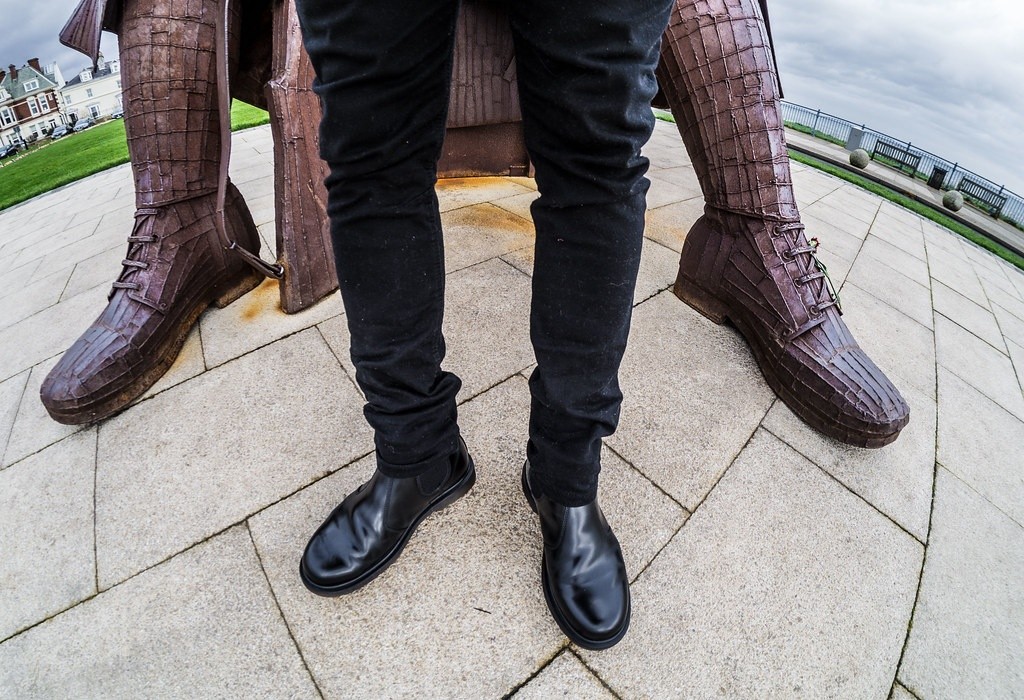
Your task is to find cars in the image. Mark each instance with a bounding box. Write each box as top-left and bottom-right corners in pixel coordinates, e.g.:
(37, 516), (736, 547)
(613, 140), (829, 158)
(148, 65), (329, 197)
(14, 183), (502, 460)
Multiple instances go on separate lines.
(0, 145), (18, 160)
(50, 124), (73, 140)
(73, 117), (96, 132)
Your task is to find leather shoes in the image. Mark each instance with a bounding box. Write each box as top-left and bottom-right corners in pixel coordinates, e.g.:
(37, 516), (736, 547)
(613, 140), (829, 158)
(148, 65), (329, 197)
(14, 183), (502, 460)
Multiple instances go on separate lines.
(299, 435), (475, 597)
(521, 460), (631, 650)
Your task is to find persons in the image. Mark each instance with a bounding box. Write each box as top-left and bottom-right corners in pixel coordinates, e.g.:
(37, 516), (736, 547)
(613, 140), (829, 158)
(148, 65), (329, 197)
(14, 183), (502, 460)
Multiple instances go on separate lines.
(38, 0), (912, 450)
(290, 0), (679, 653)
